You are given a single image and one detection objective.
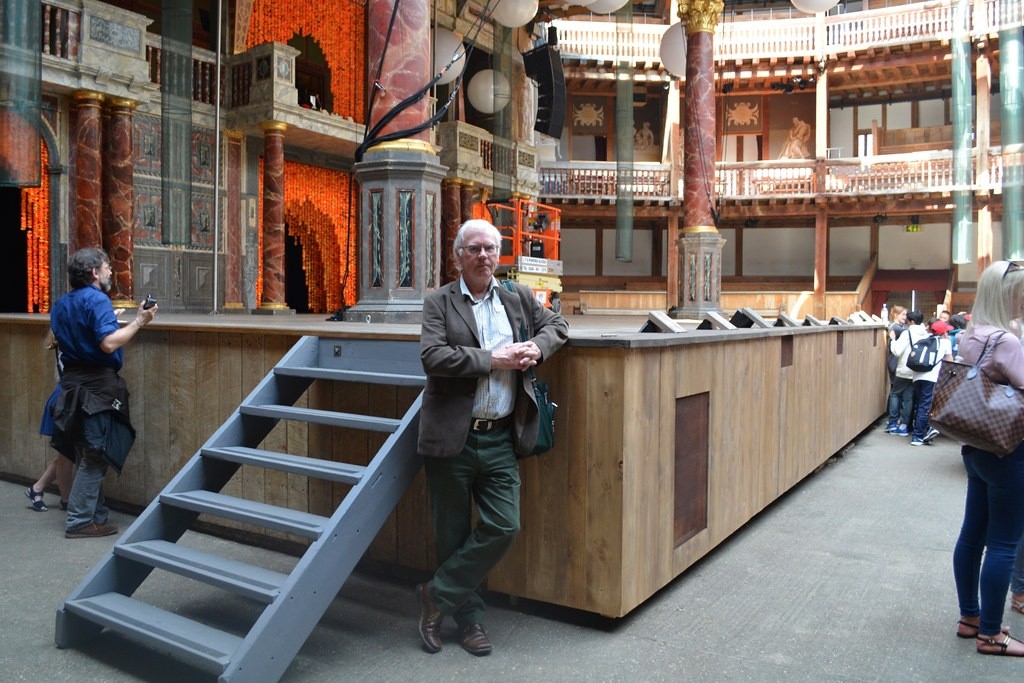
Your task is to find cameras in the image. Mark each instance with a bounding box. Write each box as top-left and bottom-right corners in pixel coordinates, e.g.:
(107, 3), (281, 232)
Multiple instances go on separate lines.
(143, 294), (156, 310)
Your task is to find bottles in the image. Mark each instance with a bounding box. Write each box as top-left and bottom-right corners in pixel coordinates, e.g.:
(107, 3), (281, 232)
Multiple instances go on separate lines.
(880, 304), (889, 326)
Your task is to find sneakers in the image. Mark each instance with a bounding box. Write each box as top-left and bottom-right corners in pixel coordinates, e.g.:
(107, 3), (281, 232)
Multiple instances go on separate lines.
(65, 522), (118, 538)
(911, 427), (939, 445)
(884, 423), (909, 436)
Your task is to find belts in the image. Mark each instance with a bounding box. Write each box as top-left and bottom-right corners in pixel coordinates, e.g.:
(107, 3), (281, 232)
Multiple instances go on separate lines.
(469, 412), (513, 432)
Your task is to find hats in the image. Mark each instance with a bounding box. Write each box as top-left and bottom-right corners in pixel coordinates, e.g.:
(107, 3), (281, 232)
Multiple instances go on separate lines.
(930, 321), (954, 334)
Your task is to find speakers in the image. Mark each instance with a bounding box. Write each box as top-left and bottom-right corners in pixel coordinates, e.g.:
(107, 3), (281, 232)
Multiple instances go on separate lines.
(523, 44), (566, 140)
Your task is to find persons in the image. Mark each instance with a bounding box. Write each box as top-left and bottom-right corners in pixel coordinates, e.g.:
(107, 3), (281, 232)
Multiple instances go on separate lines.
(23, 248), (159, 539)
(952, 259), (1024, 656)
(417, 219), (569, 655)
(884, 306), (1024, 616)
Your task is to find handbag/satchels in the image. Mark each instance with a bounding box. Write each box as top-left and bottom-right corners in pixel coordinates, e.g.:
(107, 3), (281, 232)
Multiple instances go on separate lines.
(515, 385), (557, 459)
(930, 331), (1024, 459)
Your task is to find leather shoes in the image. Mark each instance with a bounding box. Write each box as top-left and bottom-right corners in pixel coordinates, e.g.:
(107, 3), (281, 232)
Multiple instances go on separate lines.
(416, 583), (442, 652)
(457, 624), (491, 652)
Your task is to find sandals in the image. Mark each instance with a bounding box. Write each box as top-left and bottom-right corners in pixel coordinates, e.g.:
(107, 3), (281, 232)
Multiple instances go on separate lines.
(25, 485), (48, 511)
(1011, 599), (1024, 614)
(956, 619), (1009, 639)
(976, 632), (1024, 657)
(59, 498), (68, 511)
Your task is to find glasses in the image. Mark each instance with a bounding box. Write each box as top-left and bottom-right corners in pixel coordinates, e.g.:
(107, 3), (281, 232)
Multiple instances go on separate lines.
(1003, 261), (1024, 280)
(100, 265), (112, 271)
(460, 246), (498, 256)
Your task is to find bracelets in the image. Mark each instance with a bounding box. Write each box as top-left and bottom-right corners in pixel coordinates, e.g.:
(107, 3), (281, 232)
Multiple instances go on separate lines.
(135, 319), (145, 328)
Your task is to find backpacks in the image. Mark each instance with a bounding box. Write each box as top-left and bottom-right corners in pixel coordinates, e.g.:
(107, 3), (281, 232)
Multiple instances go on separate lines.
(906, 335), (942, 372)
